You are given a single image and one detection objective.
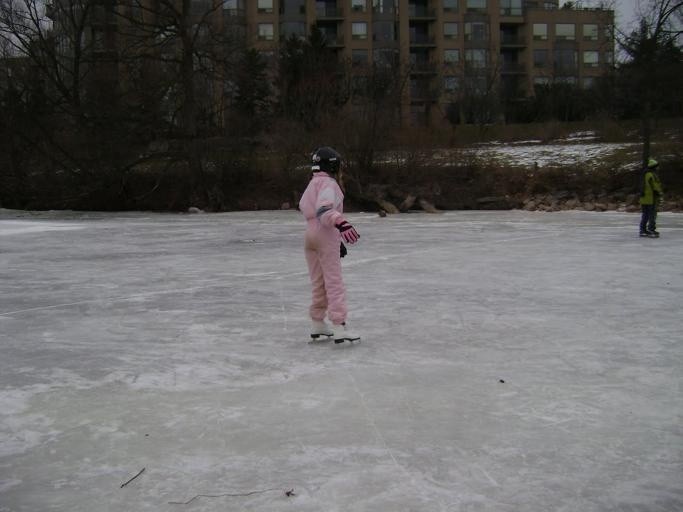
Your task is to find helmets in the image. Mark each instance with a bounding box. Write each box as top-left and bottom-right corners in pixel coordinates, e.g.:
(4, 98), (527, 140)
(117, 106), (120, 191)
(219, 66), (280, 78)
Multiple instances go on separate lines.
(311, 145), (341, 173)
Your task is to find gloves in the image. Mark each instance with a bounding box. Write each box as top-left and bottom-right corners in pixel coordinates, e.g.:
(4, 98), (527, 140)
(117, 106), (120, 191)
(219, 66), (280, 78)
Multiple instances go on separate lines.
(339, 242), (346, 257)
(334, 217), (360, 244)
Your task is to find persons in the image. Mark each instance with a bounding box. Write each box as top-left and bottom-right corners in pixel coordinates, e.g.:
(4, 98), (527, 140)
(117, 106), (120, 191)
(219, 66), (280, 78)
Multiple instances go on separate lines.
(637, 160), (663, 238)
(296, 146), (363, 344)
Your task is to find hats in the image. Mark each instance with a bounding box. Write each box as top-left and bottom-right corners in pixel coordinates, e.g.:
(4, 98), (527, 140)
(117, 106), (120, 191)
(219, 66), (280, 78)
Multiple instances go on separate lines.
(647, 159), (658, 168)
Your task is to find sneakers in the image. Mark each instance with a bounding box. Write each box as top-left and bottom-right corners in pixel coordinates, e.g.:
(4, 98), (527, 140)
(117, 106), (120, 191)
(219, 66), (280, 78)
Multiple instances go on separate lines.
(310, 320), (334, 338)
(639, 230), (649, 234)
(648, 231), (658, 235)
(332, 324), (360, 344)
